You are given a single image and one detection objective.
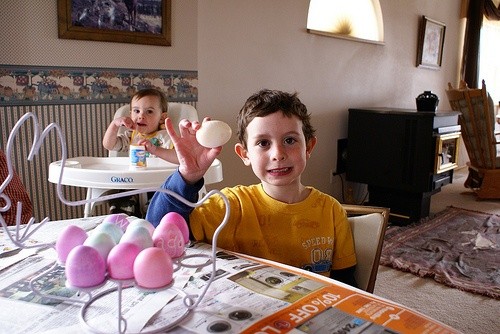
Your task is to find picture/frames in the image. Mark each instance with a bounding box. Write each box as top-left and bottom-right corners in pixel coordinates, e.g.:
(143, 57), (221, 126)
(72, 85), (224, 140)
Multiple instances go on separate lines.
(58, 0), (172, 47)
(416, 15), (447, 71)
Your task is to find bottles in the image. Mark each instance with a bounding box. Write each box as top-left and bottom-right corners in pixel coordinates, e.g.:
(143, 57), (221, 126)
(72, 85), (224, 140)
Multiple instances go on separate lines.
(416, 91), (440, 112)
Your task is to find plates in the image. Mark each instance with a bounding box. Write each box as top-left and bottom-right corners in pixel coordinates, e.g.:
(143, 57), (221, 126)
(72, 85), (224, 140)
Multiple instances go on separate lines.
(57, 160), (79, 166)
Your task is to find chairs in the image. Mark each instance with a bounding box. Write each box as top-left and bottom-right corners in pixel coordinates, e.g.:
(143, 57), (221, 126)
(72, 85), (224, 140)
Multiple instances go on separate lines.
(343, 202), (390, 295)
(107, 99), (202, 218)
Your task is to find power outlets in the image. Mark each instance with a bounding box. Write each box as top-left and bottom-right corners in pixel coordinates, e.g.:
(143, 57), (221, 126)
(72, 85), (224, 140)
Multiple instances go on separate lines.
(330, 168), (338, 184)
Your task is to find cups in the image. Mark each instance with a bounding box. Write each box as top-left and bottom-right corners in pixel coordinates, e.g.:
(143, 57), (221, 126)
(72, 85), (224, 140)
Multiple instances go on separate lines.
(130, 144), (146, 166)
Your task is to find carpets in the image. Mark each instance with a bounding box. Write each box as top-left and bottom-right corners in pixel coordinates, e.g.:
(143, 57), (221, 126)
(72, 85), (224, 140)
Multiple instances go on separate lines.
(377, 205), (500, 308)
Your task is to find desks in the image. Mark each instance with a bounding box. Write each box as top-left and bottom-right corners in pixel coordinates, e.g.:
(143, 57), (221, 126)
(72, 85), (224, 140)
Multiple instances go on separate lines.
(0, 216), (457, 334)
(348, 107), (462, 227)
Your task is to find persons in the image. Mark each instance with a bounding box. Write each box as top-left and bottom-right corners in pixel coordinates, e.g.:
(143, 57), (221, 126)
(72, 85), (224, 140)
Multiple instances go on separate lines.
(103, 89), (173, 219)
(145, 89), (357, 288)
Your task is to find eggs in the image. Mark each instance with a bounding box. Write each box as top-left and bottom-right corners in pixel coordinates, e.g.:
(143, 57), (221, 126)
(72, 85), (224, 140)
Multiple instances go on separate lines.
(196, 120), (233, 148)
(57, 212), (189, 289)
